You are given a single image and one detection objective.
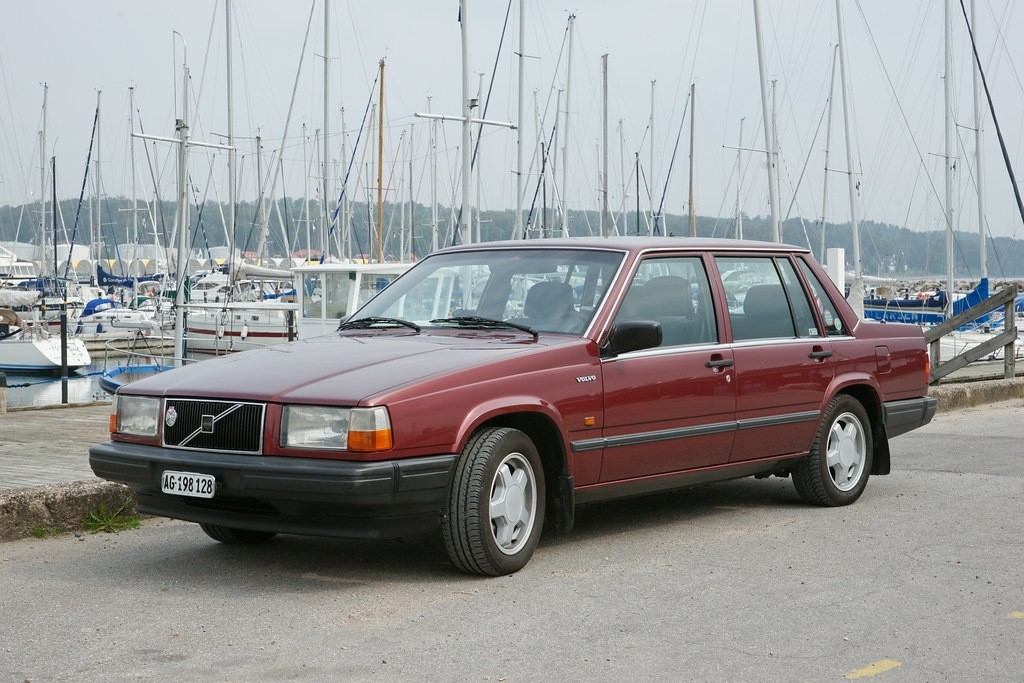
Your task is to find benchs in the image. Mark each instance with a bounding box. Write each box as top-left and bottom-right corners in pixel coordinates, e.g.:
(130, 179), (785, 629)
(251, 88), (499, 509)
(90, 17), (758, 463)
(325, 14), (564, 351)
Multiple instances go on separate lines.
(603, 280), (808, 337)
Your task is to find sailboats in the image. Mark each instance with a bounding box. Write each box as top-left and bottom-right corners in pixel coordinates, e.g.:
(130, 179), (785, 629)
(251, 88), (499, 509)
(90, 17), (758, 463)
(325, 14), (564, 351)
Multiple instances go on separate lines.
(1, 1), (1024, 403)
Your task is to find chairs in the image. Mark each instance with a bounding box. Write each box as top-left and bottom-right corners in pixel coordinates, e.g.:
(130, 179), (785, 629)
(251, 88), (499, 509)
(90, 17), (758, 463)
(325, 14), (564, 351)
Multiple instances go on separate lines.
(635, 275), (695, 346)
(525, 281), (579, 332)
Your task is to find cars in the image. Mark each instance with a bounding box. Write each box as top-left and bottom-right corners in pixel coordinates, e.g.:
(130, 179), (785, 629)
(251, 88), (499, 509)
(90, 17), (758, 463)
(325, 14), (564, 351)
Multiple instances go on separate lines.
(88, 236), (940, 581)
